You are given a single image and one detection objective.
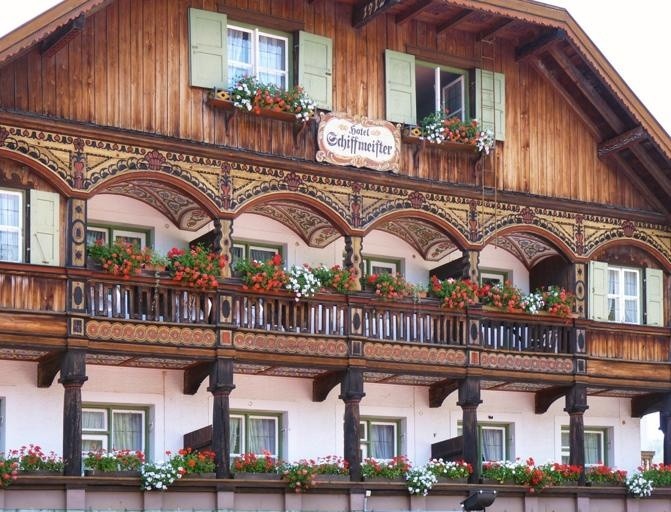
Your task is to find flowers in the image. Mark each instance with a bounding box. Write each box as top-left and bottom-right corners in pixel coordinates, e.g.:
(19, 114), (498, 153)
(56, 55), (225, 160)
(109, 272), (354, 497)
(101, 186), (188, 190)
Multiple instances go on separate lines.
(230, 73), (317, 126)
(84, 238), (576, 323)
(0, 444), (671, 498)
(401, 107), (496, 156)
